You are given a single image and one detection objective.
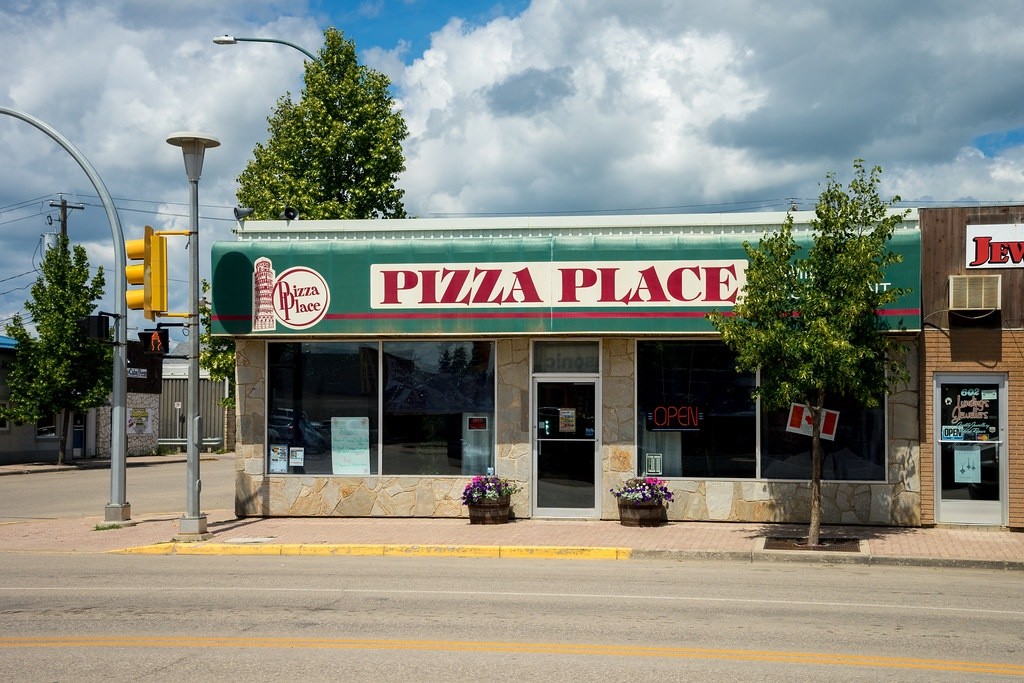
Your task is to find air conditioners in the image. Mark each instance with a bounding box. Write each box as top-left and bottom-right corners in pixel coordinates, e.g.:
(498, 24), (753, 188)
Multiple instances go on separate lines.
(947, 274), (1002, 312)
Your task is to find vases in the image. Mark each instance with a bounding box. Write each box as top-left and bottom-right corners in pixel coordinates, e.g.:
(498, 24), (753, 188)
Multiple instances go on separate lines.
(616, 496), (669, 528)
(468, 495), (511, 525)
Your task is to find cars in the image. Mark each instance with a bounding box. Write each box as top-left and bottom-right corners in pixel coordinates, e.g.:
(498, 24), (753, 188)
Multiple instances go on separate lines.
(538, 407), (595, 438)
(267, 414), (329, 457)
(37, 426), (87, 449)
(941, 438), (1002, 498)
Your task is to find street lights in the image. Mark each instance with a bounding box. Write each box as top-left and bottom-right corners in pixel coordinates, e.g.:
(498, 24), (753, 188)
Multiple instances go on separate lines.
(163, 133), (222, 547)
(214, 34), (356, 215)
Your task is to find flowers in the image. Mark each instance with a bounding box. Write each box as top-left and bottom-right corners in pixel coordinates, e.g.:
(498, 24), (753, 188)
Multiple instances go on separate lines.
(459, 472), (524, 506)
(608, 475), (675, 505)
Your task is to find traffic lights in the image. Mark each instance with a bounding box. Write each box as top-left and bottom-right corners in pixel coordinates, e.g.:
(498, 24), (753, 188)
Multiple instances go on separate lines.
(124, 224), (169, 320)
(138, 331), (169, 354)
